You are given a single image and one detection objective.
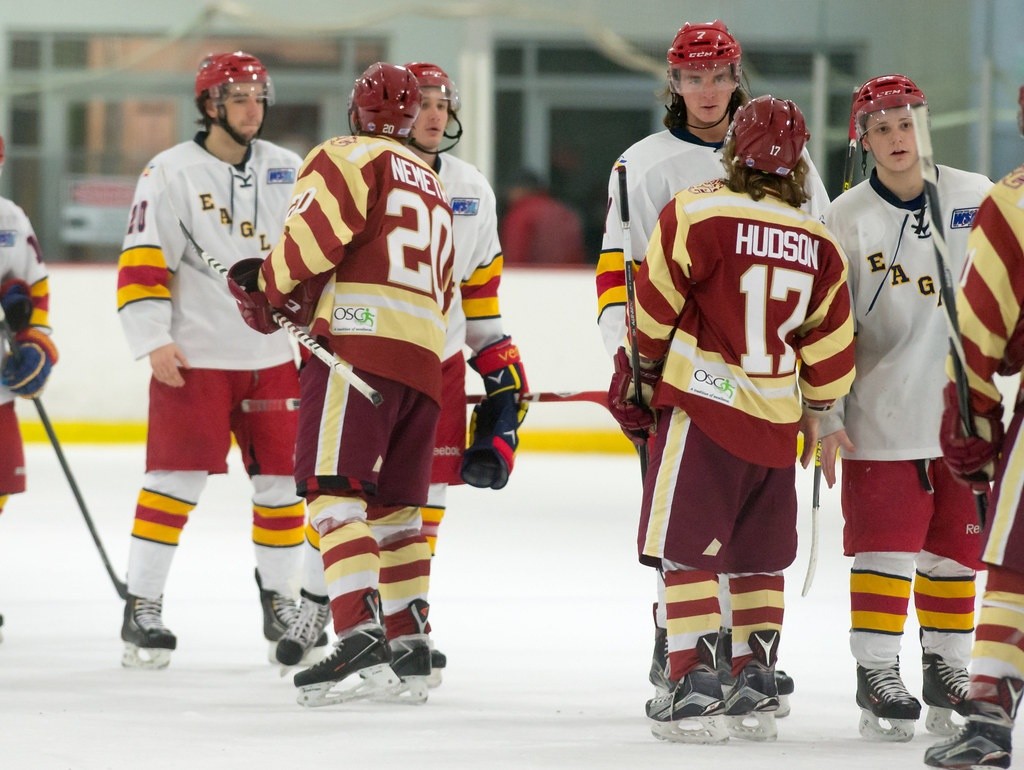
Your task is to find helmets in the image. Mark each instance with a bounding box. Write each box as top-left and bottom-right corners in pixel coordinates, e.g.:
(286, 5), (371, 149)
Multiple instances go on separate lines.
(407, 62), (452, 96)
(667, 19), (741, 70)
(853, 74), (925, 134)
(733, 94), (810, 176)
(350, 62), (422, 138)
(197, 51), (268, 97)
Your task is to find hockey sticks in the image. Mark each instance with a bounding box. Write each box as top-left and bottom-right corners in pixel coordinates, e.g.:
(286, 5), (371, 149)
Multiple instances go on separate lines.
(0, 308), (128, 599)
(842, 84), (862, 192)
(910, 104), (986, 532)
(466, 390), (610, 415)
(157, 162), (383, 408)
(801, 441), (824, 598)
(617, 166), (649, 485)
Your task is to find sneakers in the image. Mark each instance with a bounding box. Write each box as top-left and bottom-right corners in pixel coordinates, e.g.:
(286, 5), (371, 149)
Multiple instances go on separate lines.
(723, 630), (780, 742)
(120, 593), (176, 670)
(254, 567), (327, 647)
(276, 587), (331, 665)
(645, 633), (728, 744)
(648, 602), (678, 691)
(716, 627), (794, 718)
(924, 676), (1024, 770)
(389, 598), (432, 705)
(855, 656), (921, 741)
(919, 628), (971, 736)
(293, 591), (410, 706)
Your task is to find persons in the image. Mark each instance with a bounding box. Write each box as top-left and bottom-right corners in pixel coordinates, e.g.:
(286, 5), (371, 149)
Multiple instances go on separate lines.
(115, 50), (330, 668)
(0, 138), (58, 645)
(275, 62), (529, 689)
(229, 62), (453, 707)
(925, 83), (1024, 770)
(595, 18), (858, 746)
(818, 74), (998, 742)
(497, 166), (587, 268)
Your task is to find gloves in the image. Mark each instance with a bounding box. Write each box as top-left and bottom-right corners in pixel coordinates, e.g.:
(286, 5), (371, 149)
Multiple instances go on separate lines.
(467, 336), (529, 425)
(939, 382), (1004, 491)
(227, 258), (283, 334)
(1, 344), (51, 400)
(462, 394), (518, 491)
(609, 347), (660, 446)
